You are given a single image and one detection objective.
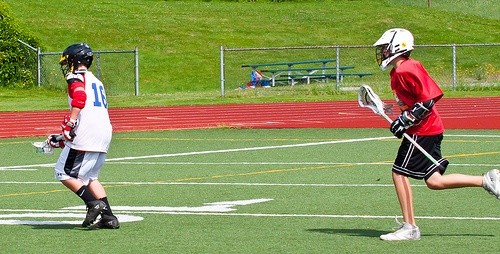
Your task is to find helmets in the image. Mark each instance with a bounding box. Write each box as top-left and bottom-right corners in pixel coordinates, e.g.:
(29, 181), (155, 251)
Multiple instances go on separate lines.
(373, 28), (414, 71)
(60, 44), (93, 78)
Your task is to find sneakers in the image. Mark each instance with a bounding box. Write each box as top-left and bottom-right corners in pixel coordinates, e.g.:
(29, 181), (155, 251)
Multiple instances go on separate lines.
(82, 201), (108, 227)
(96, 216), (119, 229)
(489, 169), (500, 200)
(380, 222), (420, 240)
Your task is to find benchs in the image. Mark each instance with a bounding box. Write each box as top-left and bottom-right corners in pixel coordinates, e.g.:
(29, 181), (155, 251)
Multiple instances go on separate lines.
(241, 59), (374, 87)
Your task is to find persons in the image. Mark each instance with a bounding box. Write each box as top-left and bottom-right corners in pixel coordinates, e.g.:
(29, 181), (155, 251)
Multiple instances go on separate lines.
(47, 42), (120, 230)
(373, 28), (500, 241)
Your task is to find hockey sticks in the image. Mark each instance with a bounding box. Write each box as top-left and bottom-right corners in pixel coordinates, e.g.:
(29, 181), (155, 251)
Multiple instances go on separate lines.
(31, 131), (76, 154)
(357, 84), (444, 170)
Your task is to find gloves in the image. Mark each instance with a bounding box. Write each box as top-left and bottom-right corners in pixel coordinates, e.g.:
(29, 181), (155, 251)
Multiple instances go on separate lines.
(390, 110), (414, 139)
(47, 134), (65, 148)
(61, 114), (80, 142)
